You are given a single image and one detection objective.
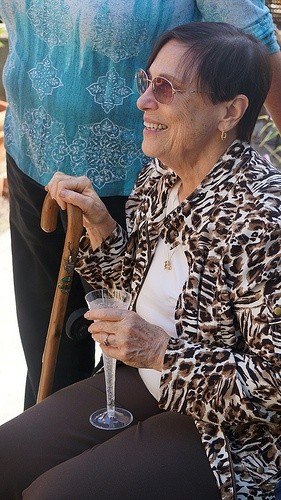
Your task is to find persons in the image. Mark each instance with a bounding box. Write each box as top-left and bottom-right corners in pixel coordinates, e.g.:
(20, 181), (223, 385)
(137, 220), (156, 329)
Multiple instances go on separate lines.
(1, 19), (280, 500)
(1, 0), (281, 415)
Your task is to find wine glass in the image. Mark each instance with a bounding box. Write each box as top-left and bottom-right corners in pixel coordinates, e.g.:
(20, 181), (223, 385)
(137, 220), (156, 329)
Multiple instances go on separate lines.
(84, 288), (133, 430)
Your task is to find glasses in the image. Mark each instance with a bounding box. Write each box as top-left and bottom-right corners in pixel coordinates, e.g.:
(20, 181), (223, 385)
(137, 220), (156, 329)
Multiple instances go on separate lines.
(135, 68), (216, 106)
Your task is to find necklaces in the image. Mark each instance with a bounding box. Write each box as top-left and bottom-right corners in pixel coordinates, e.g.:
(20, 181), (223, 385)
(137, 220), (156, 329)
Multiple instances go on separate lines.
(164, 243), (176, 270)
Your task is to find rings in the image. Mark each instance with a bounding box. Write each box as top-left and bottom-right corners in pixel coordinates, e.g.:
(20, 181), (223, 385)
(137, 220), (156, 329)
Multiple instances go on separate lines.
(104, 335), (110, 348)
